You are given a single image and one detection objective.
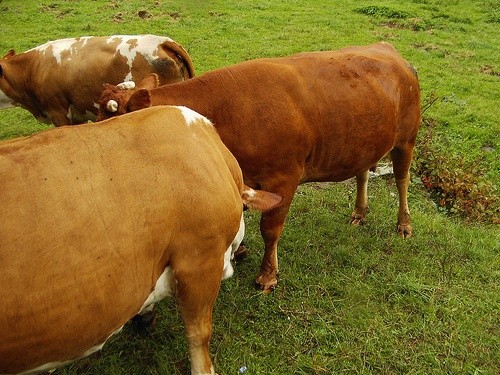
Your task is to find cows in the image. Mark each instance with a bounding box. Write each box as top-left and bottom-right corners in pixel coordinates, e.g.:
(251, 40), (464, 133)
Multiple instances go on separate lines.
(0, 33), (195, 127)
(0, 105), (284, 375)
(95, 41), (422, 294)
